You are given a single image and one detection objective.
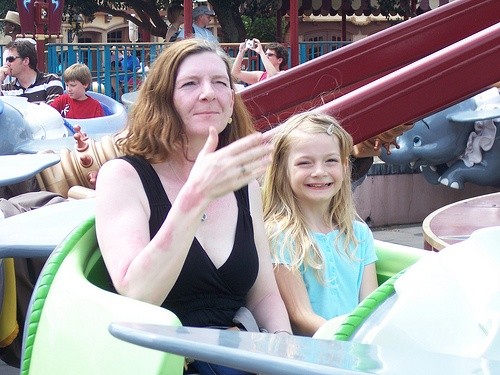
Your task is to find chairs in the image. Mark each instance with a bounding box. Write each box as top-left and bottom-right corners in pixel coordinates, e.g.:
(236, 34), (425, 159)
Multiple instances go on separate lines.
(120, 92), (139, 110)
(60, 90), (128, 137)
(20, 215), (436, 375)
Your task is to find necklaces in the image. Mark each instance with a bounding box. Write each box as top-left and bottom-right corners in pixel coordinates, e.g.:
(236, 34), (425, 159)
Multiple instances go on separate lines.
(166, 157), (207, 221)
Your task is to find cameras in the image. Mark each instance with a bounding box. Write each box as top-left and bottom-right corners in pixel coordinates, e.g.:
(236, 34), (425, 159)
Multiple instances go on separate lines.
(246, 39), (255, 48)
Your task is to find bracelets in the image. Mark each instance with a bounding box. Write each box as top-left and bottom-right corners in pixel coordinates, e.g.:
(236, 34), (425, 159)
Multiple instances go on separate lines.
(272, 330), (292, 336)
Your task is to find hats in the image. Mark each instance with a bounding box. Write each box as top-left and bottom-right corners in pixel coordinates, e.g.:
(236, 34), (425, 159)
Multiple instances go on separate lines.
(192, 5), (214, 19)
(0, 11), (21, 25)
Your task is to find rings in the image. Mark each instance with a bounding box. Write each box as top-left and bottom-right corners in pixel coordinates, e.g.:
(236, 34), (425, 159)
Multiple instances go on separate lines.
(241, 164), (248, 176)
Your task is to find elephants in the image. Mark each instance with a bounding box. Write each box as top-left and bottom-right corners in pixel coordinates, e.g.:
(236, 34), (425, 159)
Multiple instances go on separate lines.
(380, 86), (500, 191)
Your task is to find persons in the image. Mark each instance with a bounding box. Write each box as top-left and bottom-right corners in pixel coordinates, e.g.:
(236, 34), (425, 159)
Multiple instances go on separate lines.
(0, 5), (220, 119)
(261, 110), (379, 337)
(230, 37), (289, 85)
(94, 38), (293, 375)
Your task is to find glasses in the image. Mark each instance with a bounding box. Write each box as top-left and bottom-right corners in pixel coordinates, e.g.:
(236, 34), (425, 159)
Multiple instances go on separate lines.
(266, 52), (276, 58)
(5, 56), (23, 63)
